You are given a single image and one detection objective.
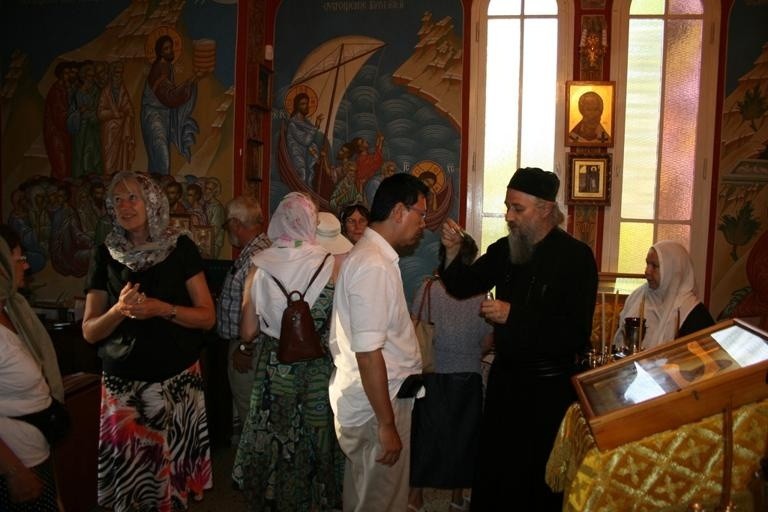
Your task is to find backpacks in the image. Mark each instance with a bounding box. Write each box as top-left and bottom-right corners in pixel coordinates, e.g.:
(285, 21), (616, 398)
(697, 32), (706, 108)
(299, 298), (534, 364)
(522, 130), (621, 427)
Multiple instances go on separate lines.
(272, 253), (332, 365)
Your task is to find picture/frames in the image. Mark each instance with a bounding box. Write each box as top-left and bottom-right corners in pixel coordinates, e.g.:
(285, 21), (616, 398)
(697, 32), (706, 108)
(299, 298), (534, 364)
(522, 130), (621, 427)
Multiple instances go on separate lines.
(563, 151), (613, 207)
(563, 80), (614, 150)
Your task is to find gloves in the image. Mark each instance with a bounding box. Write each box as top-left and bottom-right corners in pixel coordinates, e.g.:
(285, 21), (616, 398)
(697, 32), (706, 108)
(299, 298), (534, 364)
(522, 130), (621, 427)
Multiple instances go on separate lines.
(232, 349), (255, 375)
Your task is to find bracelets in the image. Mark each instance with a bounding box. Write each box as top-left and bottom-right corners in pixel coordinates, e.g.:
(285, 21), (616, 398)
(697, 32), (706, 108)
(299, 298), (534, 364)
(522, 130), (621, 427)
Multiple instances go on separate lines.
(166, 304), (178, 322)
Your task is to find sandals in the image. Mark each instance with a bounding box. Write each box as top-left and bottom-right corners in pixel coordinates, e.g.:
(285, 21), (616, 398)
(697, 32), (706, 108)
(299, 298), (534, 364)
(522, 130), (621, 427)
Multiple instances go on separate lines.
(448, 496), (471, 512)
(408, 504), (437, 512)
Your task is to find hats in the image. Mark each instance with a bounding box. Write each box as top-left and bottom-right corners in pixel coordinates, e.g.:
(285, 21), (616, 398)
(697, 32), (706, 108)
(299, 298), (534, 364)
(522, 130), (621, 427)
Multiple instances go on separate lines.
(314, 211), (354, 256)
(508, 167), (560, 203)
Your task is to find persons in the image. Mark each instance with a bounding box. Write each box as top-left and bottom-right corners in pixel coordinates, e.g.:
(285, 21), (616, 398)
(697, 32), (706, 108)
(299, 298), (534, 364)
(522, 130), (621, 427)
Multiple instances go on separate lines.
(284, 91), (436, 213)
(435, 167), (602, 512)
(612, 240), (718, 353)
(568, 92), (611, 142)
(80, 169), (216, 511)
(0, 219), (70, 511)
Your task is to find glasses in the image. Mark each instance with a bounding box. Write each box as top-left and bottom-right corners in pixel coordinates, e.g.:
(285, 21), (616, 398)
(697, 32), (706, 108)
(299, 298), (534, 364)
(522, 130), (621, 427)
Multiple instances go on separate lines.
(16, 256), (27, 265)
(403, 204), (427, 221)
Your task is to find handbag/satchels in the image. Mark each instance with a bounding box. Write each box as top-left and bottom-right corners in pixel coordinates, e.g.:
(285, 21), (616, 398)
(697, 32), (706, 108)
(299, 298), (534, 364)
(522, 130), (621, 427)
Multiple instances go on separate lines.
(407, 278), (439, 373)
(6, 366), (71, 448)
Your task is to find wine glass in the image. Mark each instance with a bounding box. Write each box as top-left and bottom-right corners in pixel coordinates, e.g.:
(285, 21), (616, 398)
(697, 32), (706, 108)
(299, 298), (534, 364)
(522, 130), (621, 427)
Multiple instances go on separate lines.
(624, 318), (646, 353)
